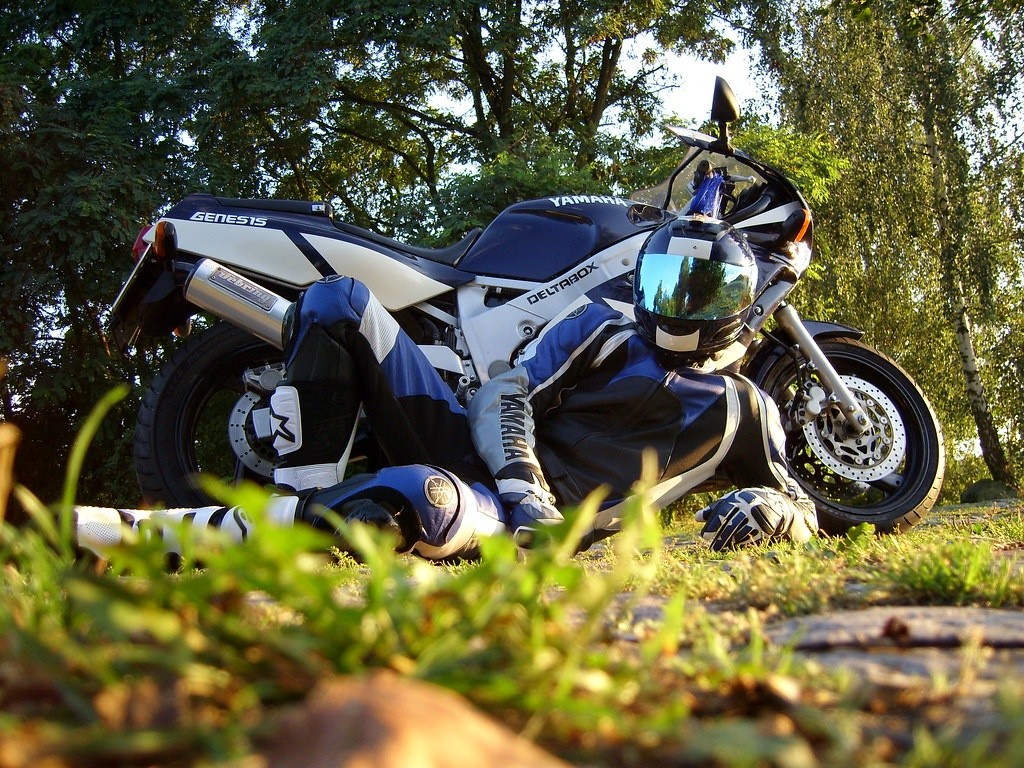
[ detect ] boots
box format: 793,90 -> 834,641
270,380 -> 363,493
71,488 -> 315,573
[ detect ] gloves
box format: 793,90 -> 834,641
695,487 -> 798,553
497,467 -> 565,541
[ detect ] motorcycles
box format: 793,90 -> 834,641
104,76 -> 947,536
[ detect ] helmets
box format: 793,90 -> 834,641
633,214 -> 758,365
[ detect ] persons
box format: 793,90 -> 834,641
48,216 -> 822,559
